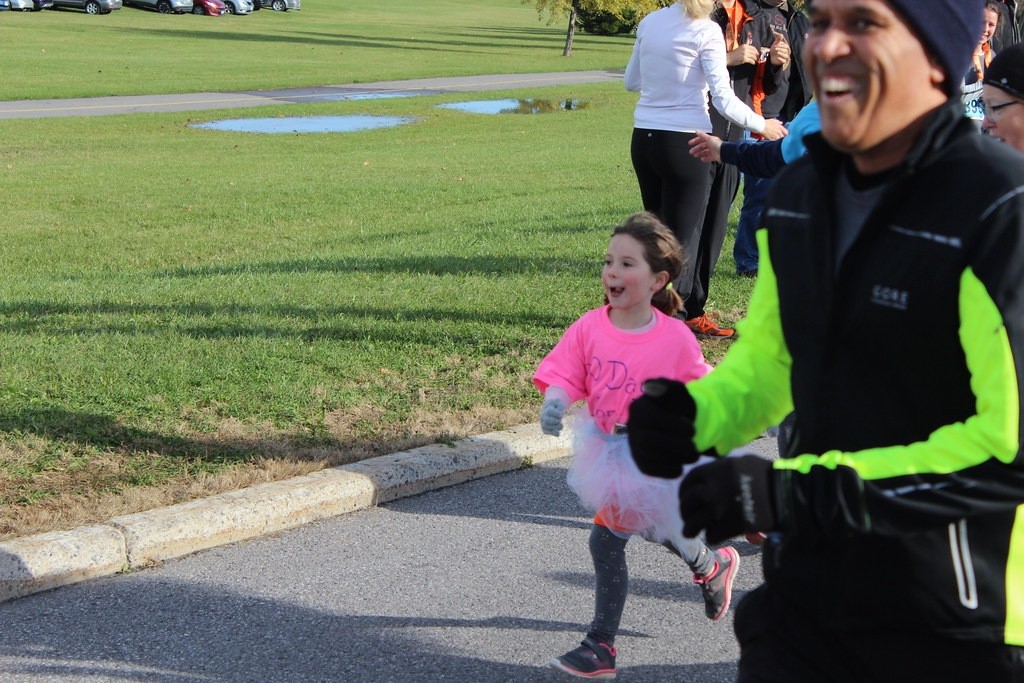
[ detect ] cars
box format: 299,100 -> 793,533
193,0 -> 227,16
0,0 -> 55,11
126,0 -> 194,13
259,0 -> 302,12
224,0 -> 254,15
56,0 -> 123,14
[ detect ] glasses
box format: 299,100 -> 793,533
975,98 -> 1017,118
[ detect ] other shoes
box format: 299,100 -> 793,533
736,268 -> 757,279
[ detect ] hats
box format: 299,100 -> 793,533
885,1 -> 983,98
983,44 -> 1024,100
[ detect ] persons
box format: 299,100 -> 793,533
533,213 -> 741,679
627,0 -> 1024,683
623,0 -> 1024,337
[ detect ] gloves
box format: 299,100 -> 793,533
542,395 -> 565,436
678,456 -> 780,548
626,384 -> 698,478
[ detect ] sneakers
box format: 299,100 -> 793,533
686,314 -> 735,339
547,637 -> 617,680
694,545 -> 740,620
